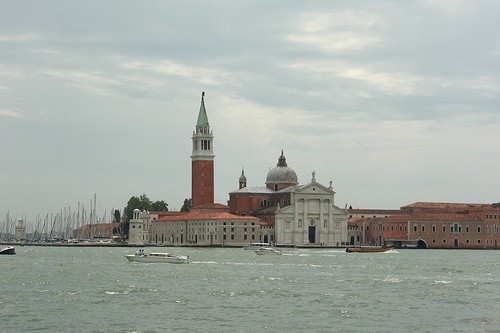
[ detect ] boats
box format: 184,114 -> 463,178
243,243 -> 273,250
345,246 -> 392,252
0,246 -> 16,255
255,248 -> 281,255
125,249 -> 190,264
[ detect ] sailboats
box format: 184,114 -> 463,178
1,193 -> 127,245
